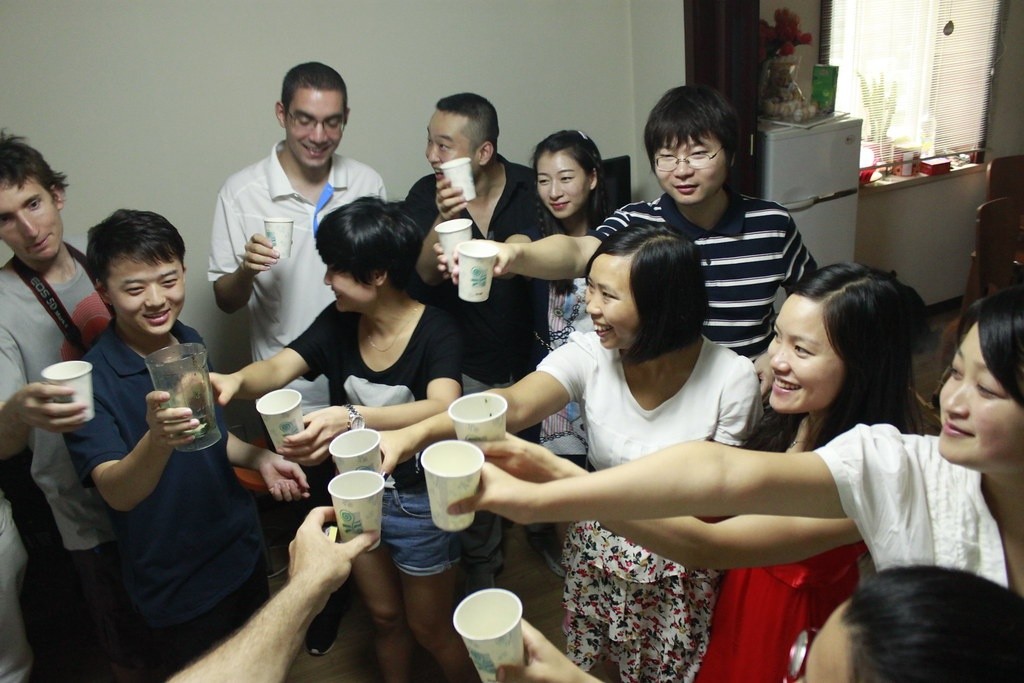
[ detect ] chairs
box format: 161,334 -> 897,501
965,157 -> 1024,311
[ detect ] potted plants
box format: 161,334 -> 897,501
850,71 -> 895,163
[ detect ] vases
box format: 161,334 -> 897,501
759,53 -> 801,110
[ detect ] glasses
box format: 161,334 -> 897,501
783,628 -> 816,683
653,145 -> 724,171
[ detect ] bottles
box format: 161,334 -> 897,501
918,109 -> 937,159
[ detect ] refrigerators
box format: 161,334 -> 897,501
757,116 -> 863,269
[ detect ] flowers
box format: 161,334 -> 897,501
759,7 -> 812,55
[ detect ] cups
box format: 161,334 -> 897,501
439,157 -> 476,203
264,218 -> 294,260
41,361 -> 95,421
143,343 -> 222,453
329,428 -> 381,475
453,589 -> 526,683
455,240 -> 499,302
255,389 -> 304,450
447,392 -> 507,446
329,470 -> 385,553
420,439 -> 485,532
434,219 -> 473,273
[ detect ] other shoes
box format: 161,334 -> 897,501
305,580 -> 350,655
528,527 -> 566,577
469,550 -> 503,593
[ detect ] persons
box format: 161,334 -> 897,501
472,261 -> 930,682
180,197 -> 481,683
0,135 -> 117,683
450,85 -> 816,433
448,286 -> 1024,594
495,566 -> 1024,683
64,208 -> 311,682
434,130 -> 612,472
162,506 -> 380,683
405,92 -> 567,577
208,62 -> 387,656
331,222 -> 763,683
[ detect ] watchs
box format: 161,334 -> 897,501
342,402 -> 365,431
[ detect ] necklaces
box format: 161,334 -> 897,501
789,417 -> 816,449
367,300 -> 419,353
